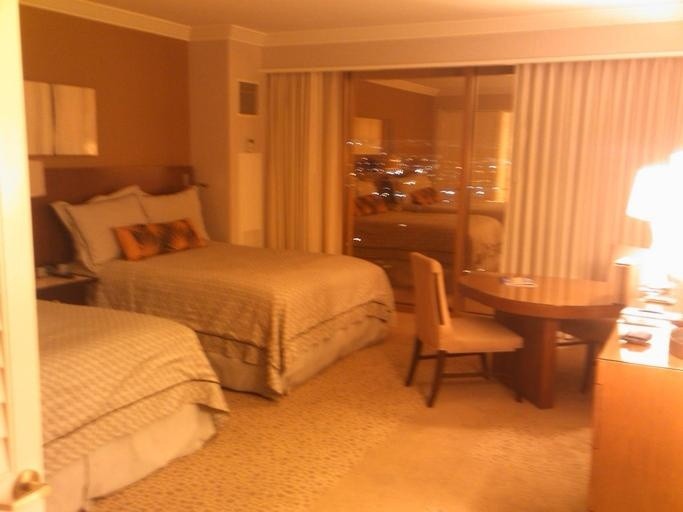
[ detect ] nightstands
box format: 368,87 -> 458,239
37,275 -> 98,305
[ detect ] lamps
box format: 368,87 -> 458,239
29,160 -> 46,198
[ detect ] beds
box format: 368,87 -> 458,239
38,299 -> 230,512
405,202 -> 503,225
355,211 -> 501,293
31,166 -> 394,402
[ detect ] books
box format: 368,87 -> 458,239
499,275 -> 538,288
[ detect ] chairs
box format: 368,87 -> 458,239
404,253 -> 526,408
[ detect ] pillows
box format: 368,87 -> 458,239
49,186 -> 210,273
355,174 -> 444,218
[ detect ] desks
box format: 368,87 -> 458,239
588,307 -> 683,512
